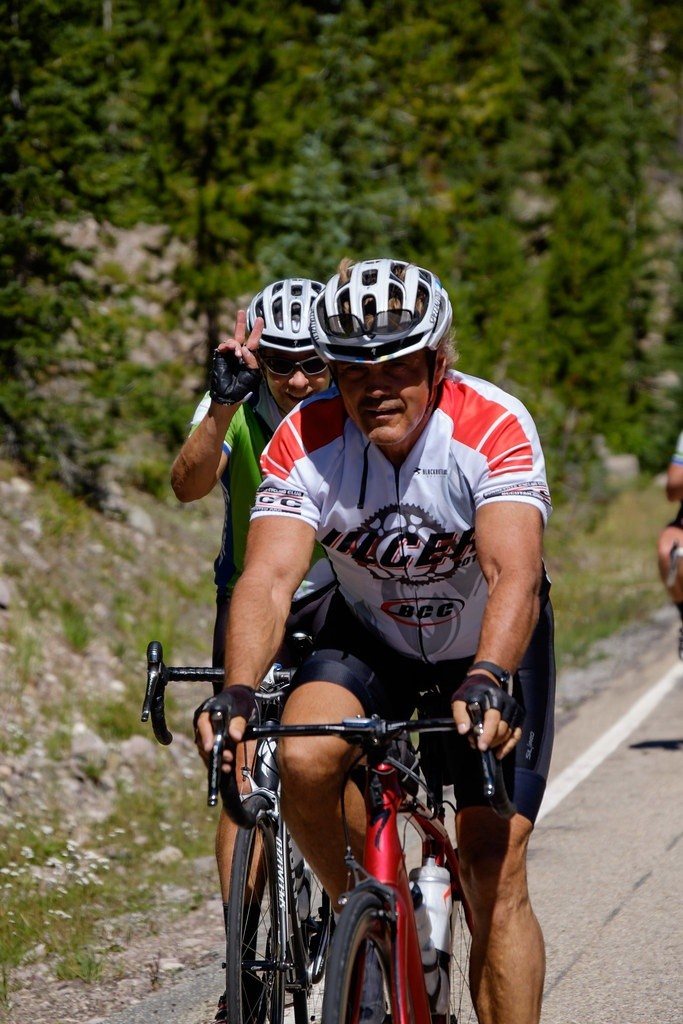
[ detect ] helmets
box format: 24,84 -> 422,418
245,277 -> 326,353
309,258 -> 453,373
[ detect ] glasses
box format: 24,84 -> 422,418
323,309 -> 417,336
260,355 -> 328,376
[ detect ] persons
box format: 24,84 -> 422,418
193,260 -> 558,1024
657,430 -> 683,659
170,279 -> 342,1024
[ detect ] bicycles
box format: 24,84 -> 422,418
138,640 -> 508,1024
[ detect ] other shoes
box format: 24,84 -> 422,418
214,975 -> 261,1024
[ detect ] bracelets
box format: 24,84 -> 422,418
469,662 -> 510,692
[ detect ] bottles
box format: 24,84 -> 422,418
406,879 -> 438,998
409,855 -> 453,1013
